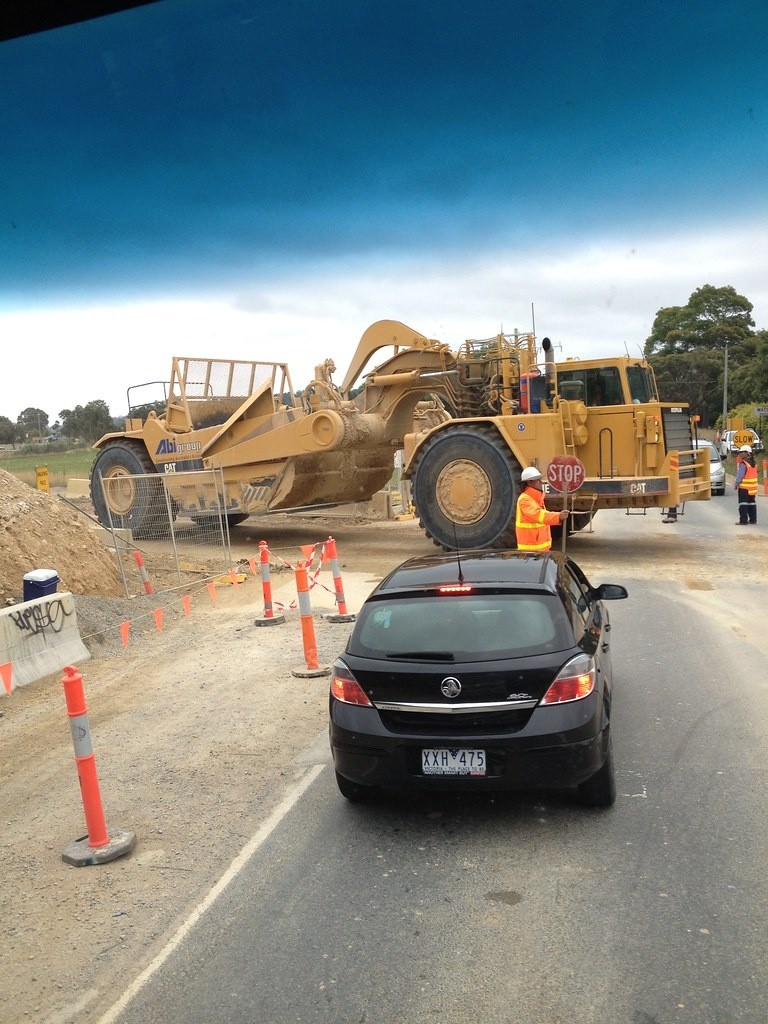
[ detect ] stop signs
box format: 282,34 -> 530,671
547,455 -> 585,494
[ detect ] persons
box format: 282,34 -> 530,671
733,445 -> 758,525
514,466 -> 570,552
662,507 -> 677,523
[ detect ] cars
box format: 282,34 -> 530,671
328,548 -> 629,811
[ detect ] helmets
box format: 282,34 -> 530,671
519,467 -> 544,484
737,445 -> 752,453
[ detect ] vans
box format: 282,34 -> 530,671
691,439 -> 728,496
721,428 -> 765,457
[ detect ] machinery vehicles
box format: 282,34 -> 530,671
88,302 -> 713,555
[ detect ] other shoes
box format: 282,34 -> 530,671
748,521 -> 757,524
735,522 -> 748,525
662,517 -> 678,523
